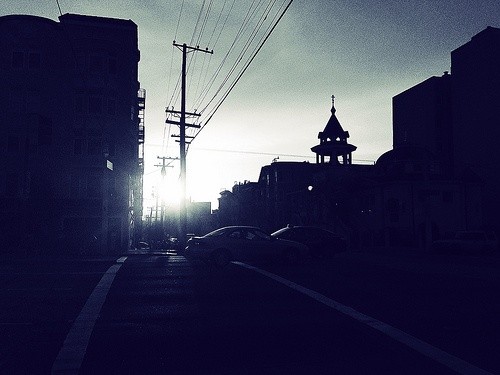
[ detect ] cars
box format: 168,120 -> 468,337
185,226 -> 308,269
134,232 -> 196,251
269,224 -> 347,257
